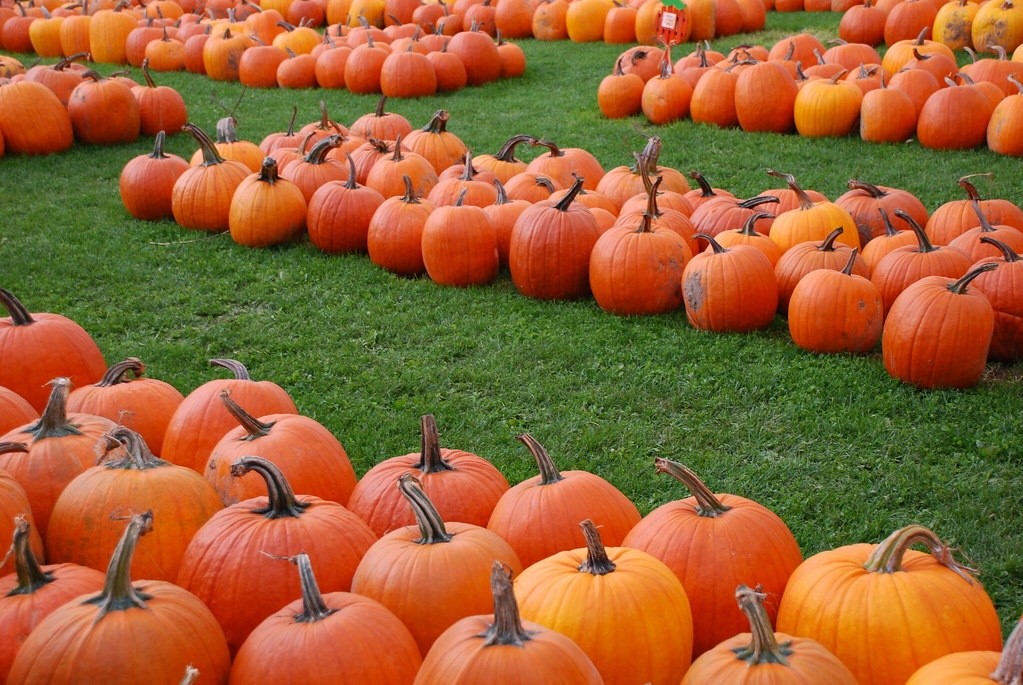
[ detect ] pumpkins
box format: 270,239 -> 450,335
0,0 -> 1023,389
0,287 -> 1023,685
